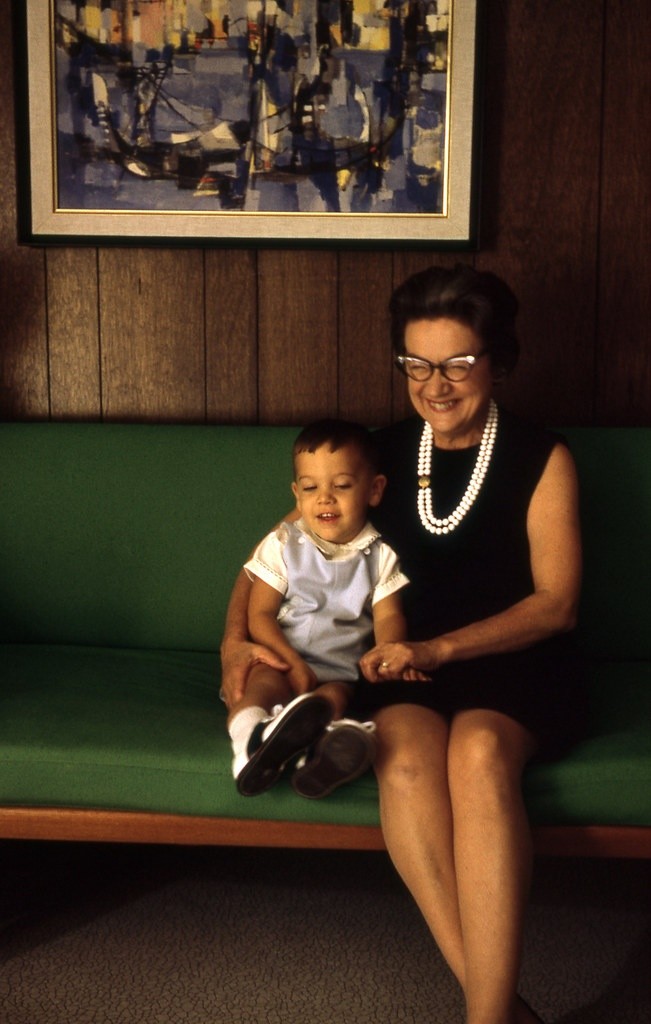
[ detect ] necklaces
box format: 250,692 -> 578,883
417,398 -> 498,535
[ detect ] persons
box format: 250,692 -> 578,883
222,267 -> 582,1024
226,415 -> 426,795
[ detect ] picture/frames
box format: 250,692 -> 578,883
9,0 -> 504,253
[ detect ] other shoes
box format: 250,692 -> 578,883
235,692 -> 334,799
290,718 -> 376,798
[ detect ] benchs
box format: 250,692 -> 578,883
0,421 -> 650,862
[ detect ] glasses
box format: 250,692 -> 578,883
394,345 -> 496,382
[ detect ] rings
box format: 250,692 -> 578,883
380,662 -> 387,666
